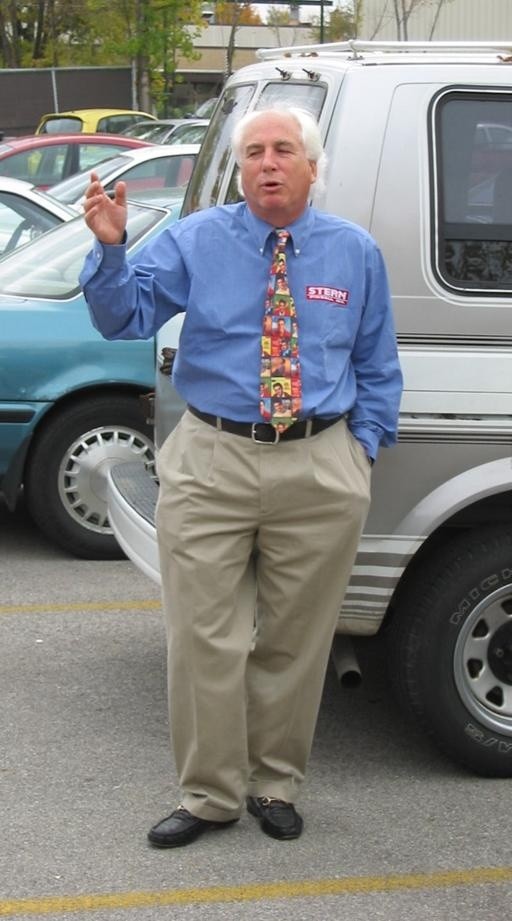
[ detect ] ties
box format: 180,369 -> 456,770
258,230 -> 301,434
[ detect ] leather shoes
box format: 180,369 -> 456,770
147,804 -> 240,848
246,796 -> 303,839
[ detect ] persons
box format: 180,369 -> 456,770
81,101 -> 404,850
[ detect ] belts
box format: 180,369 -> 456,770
187,405 -> 340,442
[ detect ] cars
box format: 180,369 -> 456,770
27,109 -> 160,179
1,174 -> 82,250
42,145 -> 205,212
183,96 -> 220,119
116,118 -> 210,148
3,184 -> 198,563
1,133 -> 198,193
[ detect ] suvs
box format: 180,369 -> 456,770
104,35 -> 511,780
